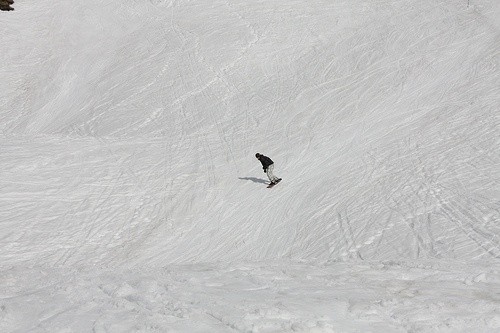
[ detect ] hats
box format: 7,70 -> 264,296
255,153 -> 260,157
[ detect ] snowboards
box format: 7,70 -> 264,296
267,178 -> 282,188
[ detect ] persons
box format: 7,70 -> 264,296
255,152 -> 281,185
